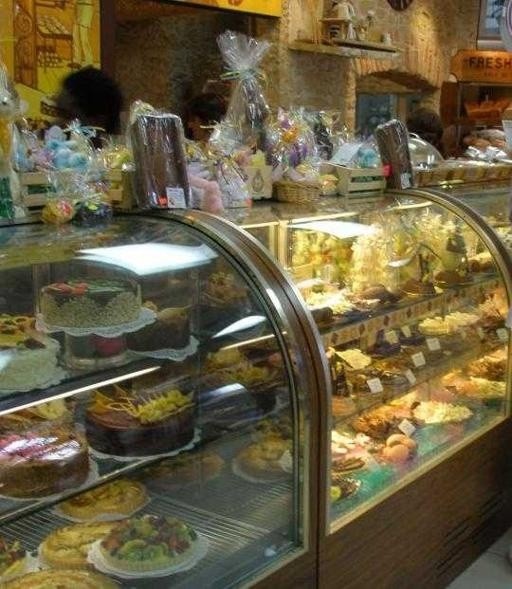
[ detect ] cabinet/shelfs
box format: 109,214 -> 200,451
1,202 -> 322,587
396,174 -> 511,416
127,186 -> 512,587
439,78 -> 512,157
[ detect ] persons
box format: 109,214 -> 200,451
403,106 -> 444,147
179,89 -> 229,141
36,64 -> 124,150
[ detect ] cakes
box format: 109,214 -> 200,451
1,538 -> 32,583
324,290 -> 508,418
329,346 -> 506,505
194,348 -> 280,431
84,382 -> 198,458
2,569 -> 122,589
86,514 -> 209,580
34,521 -> 130,570
230,433 -> 296,484
193,269 -> 259,332
141,452 -> 227,489
1,398 -> 102,498
286,231 -> 499,326
0,275 -> 199,398
52,477 -> 152,523
